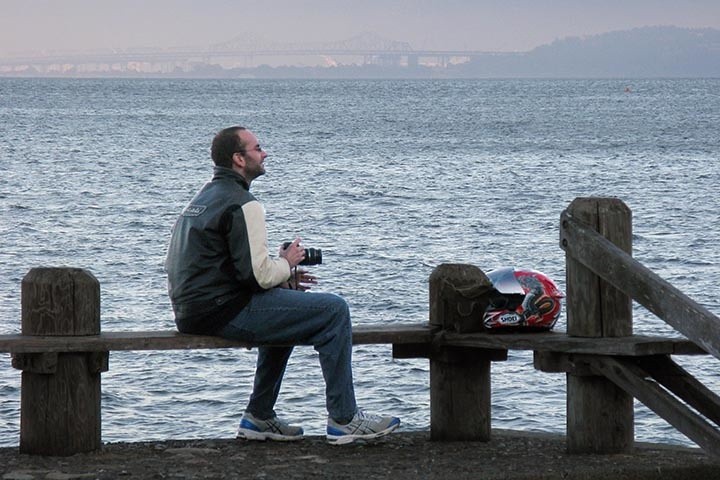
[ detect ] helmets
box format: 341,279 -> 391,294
481,266 -> 567,332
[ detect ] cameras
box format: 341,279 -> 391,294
283,242 -> 322,265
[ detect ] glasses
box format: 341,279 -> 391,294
233,145 -> 262,153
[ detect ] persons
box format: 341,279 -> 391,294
165,126 -> 400,445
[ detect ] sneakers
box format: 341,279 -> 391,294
236,413 -> 304,442
326,408 -> 400,445
